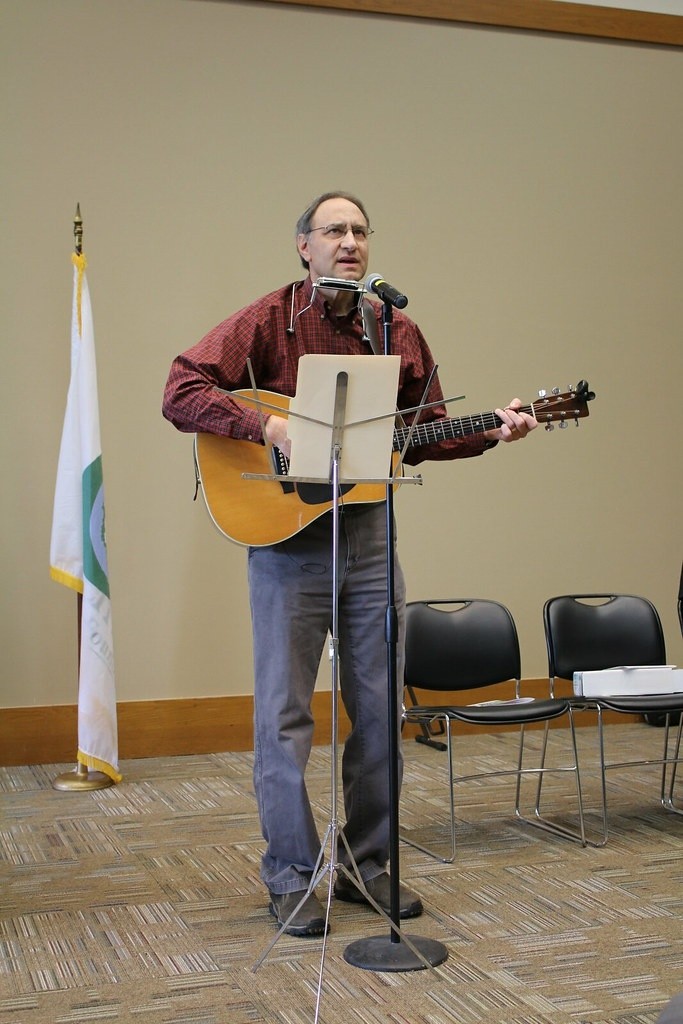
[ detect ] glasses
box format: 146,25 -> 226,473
305,223 -> 374,238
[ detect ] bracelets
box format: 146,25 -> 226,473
486,441 -> 494,447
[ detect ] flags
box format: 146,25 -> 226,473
50,252 -> 118,781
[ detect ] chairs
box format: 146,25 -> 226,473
533,592 -> 682,848
398,597 -> 588,862
666,600 -> 683,809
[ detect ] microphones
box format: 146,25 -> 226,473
364,273 -> 409,309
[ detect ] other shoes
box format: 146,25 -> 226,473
334,872 -> 423,920
268,888 -> 331,937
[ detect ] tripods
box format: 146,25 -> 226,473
238,354 -> 445,1024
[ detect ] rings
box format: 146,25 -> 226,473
512,428 -> 516,432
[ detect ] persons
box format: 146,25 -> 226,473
162,191 -> 537,936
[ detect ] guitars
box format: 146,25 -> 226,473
193,379 -> 596,549
